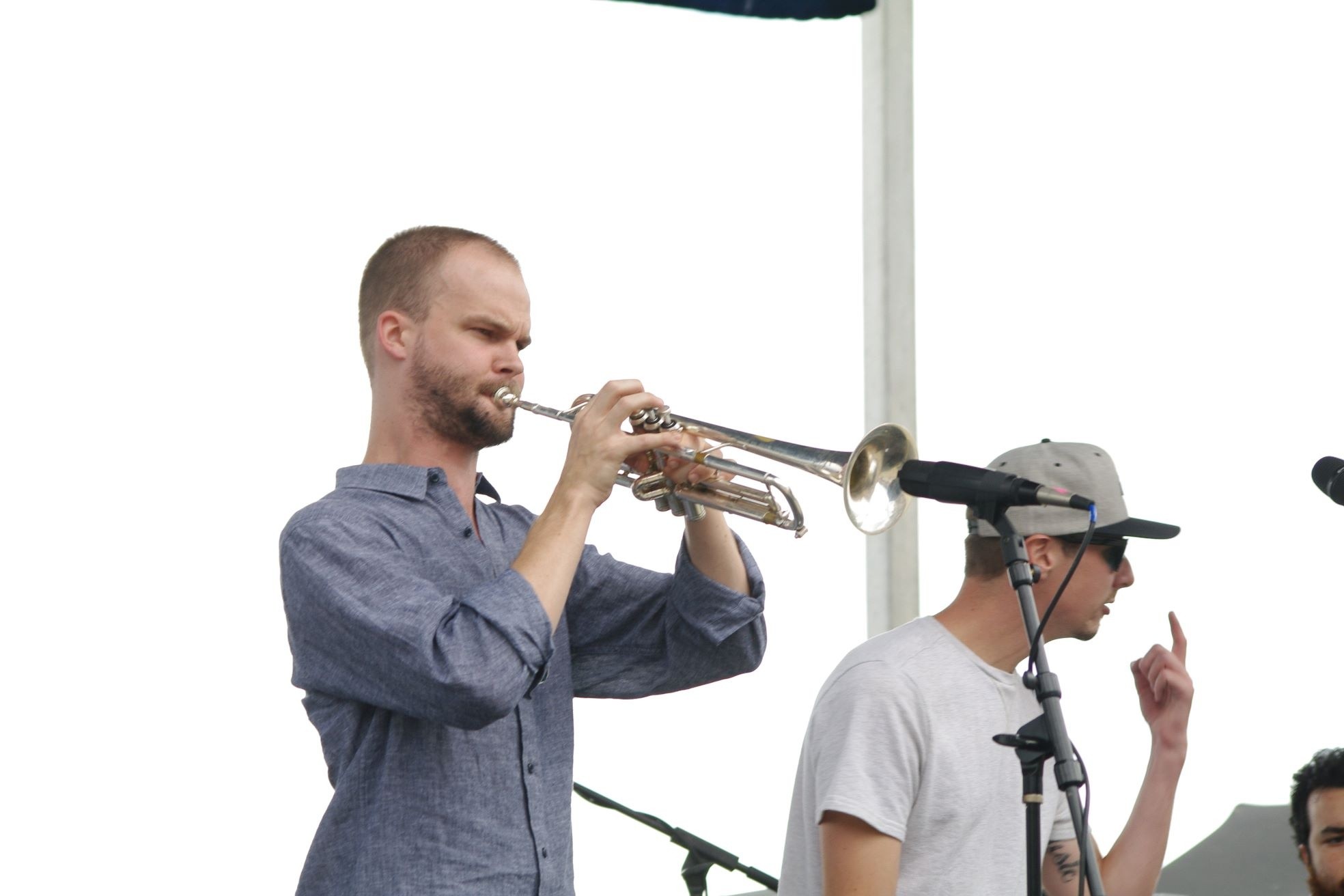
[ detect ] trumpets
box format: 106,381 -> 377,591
495,383 -> 916,538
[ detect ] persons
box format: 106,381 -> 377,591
1289,747 -> 1344,896
277,224 -> 765,896
775,437 -> 1194,896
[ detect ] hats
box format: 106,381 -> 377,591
964,439 -> 1181,540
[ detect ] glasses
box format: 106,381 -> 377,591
1061,537 -> 1129,570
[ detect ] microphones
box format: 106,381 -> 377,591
1311,456 -> 1344,506
898,459 -> 1095,511
